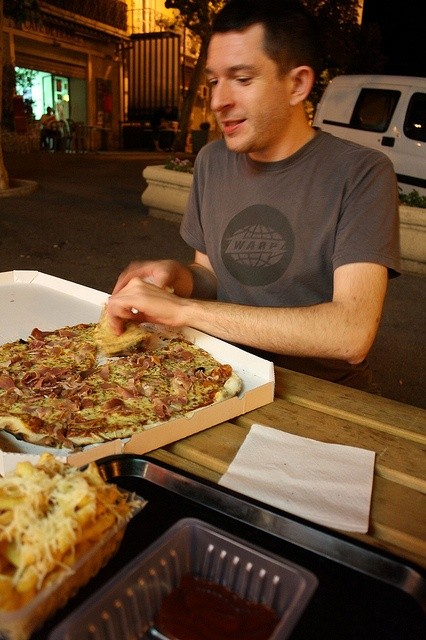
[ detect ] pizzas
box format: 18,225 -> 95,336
0,319 -> 240,447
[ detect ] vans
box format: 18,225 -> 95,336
310,73 -> 426,201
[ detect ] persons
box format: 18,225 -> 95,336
106,0 -> 403,395
40,106 -> 57,151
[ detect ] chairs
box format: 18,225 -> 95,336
47,118 -> 110,153
